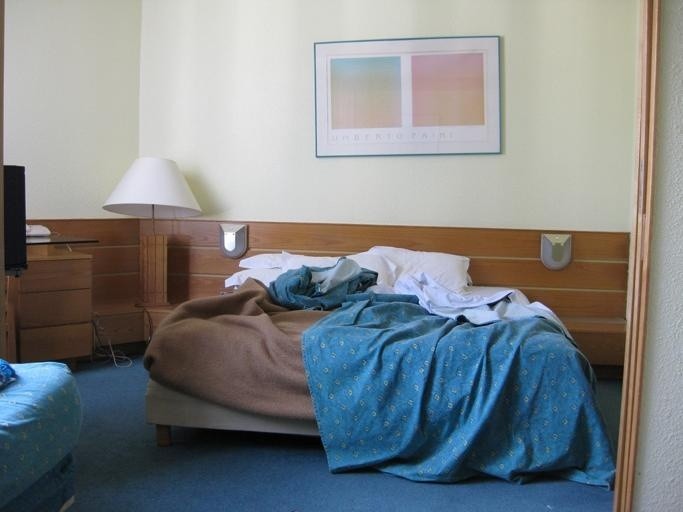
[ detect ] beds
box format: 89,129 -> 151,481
143,244 -> 622,492
0,357 -> 84,512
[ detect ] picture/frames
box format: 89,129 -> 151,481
311,34 -> 506,158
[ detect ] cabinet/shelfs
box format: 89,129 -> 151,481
13,252 -> 95,370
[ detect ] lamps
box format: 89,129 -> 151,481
101,156 -> 203,305
